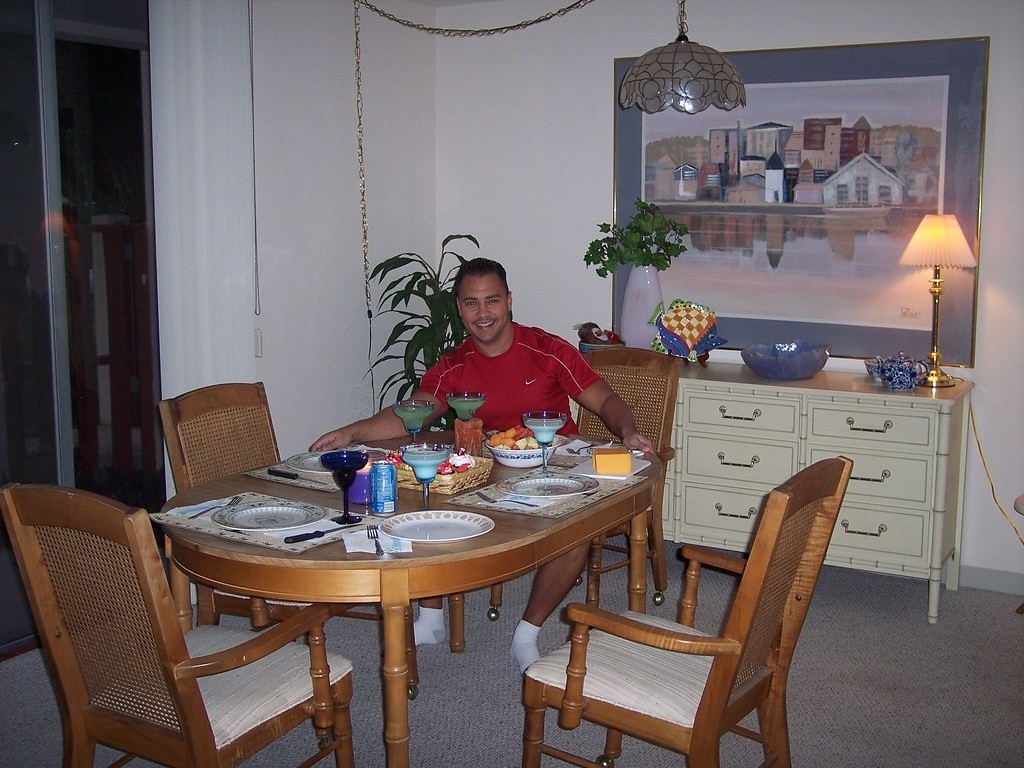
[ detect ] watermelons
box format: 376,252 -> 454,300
511,425 -> 541,450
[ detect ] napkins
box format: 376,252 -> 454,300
342,529 -> 415,555
554,439 -> 613,456
165,500 -> 227,521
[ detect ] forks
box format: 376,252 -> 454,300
566,444 -> 596,454
366,525 -> 384,556
188,496 -> 243,520
476,491 -> 540,508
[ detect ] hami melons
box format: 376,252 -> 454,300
489,427 -> 515,448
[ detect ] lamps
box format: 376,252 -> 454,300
618,2 -> 748,116
895,214 -> 978,389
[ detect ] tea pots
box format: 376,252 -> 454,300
875,351 -> 929,392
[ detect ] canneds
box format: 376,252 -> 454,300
368,460 -> 397,517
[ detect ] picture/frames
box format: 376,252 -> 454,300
611,37 -> 990,368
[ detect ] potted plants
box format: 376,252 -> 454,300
585,198 -> 689,351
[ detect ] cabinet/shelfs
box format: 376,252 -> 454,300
659,381 -> 965,581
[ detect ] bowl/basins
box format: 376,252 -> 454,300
485,435 -> 566,467
742,341 -> 832,379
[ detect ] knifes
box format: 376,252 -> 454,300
284,524 -> 365,543
268,468 -> 328,487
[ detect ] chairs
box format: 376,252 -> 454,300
0,479 -> 360,767
523,454 -> 854,768
159,381 -> 304,632
488,348 -> 683,621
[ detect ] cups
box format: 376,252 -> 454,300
445,391 -> 486,421
864,359 -> 878,379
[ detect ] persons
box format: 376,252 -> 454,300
308,258 -> 657,678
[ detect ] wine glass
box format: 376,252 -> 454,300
399,443 -> 451,511
522,410 -> 567,474
320,451 -> 368,524
391,400 -> 433,444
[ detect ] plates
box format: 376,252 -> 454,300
494,472 -> 599,497
212,500 -> 327,532
286,448 -> 385,474
580,341 -> 626,348
566,456 -> 653,480
378,510 -> 495,543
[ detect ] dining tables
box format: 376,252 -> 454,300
159,427 -> 661,767
